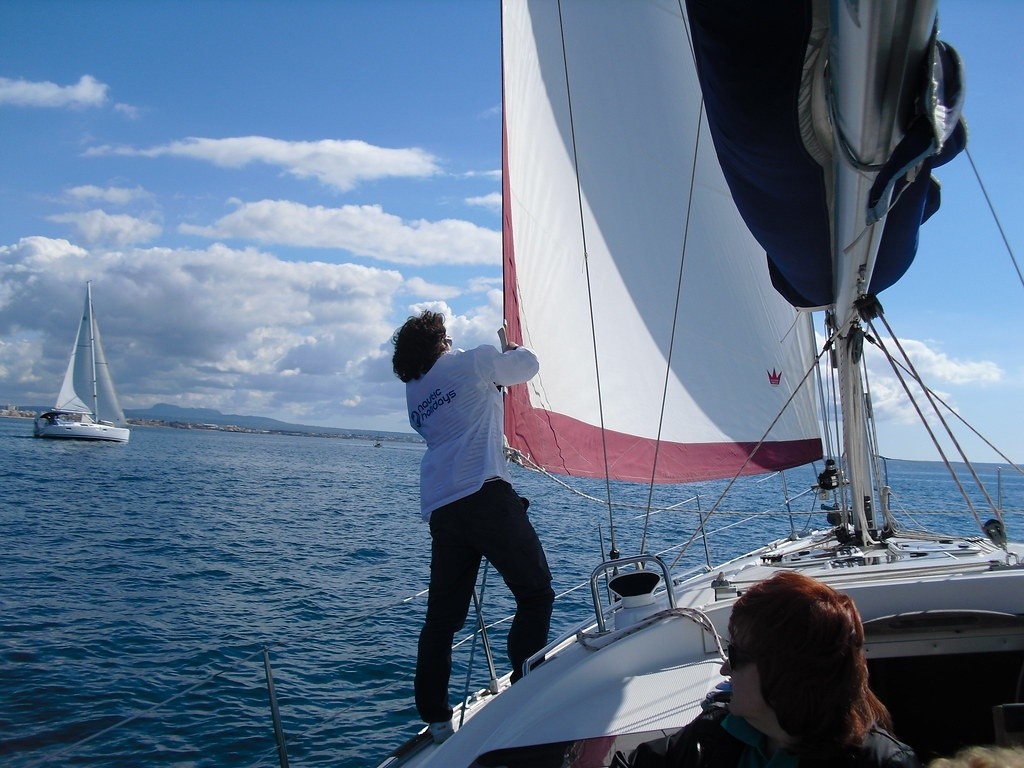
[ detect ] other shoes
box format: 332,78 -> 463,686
428,721 -> 455,744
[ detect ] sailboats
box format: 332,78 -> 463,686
33,280 -> 131,443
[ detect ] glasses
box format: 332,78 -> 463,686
441,335 -> 453,348
725,645 -> 776,673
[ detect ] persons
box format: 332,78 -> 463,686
613,570 -> 923,768
394,311 -> 558,745
